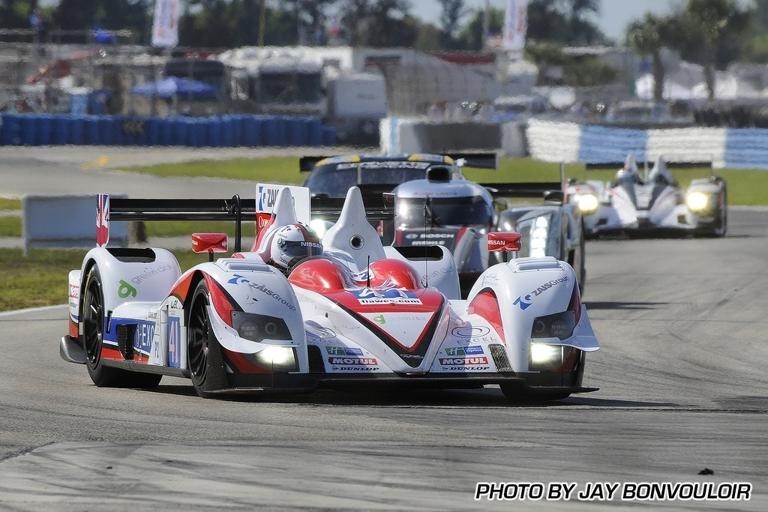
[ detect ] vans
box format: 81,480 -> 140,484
84,46 -> 389,144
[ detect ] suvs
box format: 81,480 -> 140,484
486,92 -> 550,115
600,94 -> 670,124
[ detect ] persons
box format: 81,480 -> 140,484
614,167 -> 638,184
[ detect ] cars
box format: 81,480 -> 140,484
556,156 -> 729,242
